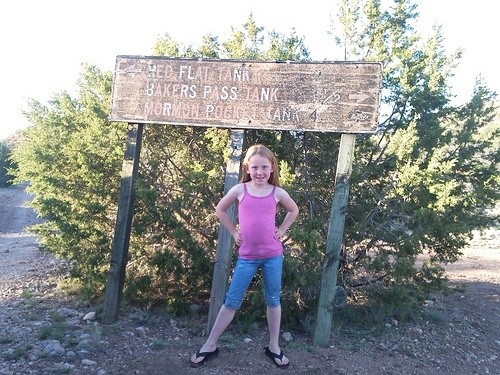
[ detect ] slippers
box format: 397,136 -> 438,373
190,348 -> 218,368
266,347 -> 290,368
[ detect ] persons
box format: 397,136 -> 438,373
190,144 -> 300,366
